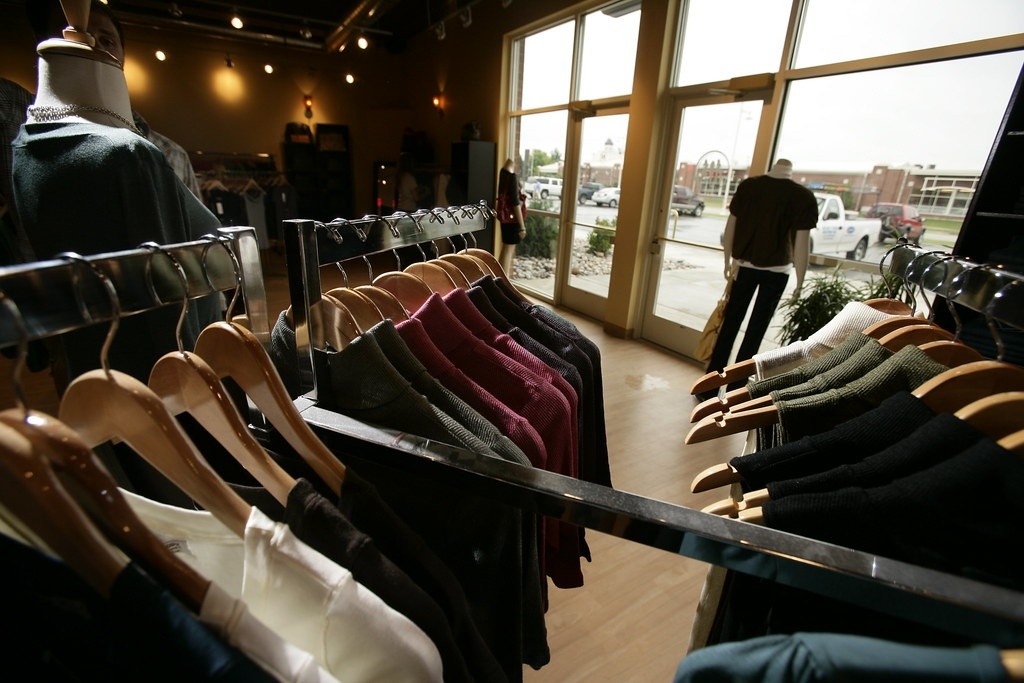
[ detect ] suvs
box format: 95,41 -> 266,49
868,202 -> 928,248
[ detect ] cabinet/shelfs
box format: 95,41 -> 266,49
450,139 -> 497,259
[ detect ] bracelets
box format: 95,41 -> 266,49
520,228 -> 527,231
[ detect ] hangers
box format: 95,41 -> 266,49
0,166 -> 601,683
676,242 -> 1024,683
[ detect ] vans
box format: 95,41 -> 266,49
524,175 -> 563,201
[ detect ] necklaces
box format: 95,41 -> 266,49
27,105 -> 149,138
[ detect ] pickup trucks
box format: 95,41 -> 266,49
805,191 -> 883,263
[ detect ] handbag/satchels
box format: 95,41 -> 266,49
692,271 -> 734,361
497,173 -> 528,225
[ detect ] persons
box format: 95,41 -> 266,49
497,158 -> 529,274
12,39 -> 222,401
85,0 -> 203,204
695,158 -> 818,403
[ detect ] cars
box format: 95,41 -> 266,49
592,187 -> 621,209
577,181 -> 605,205
670,184 -> 705,218
